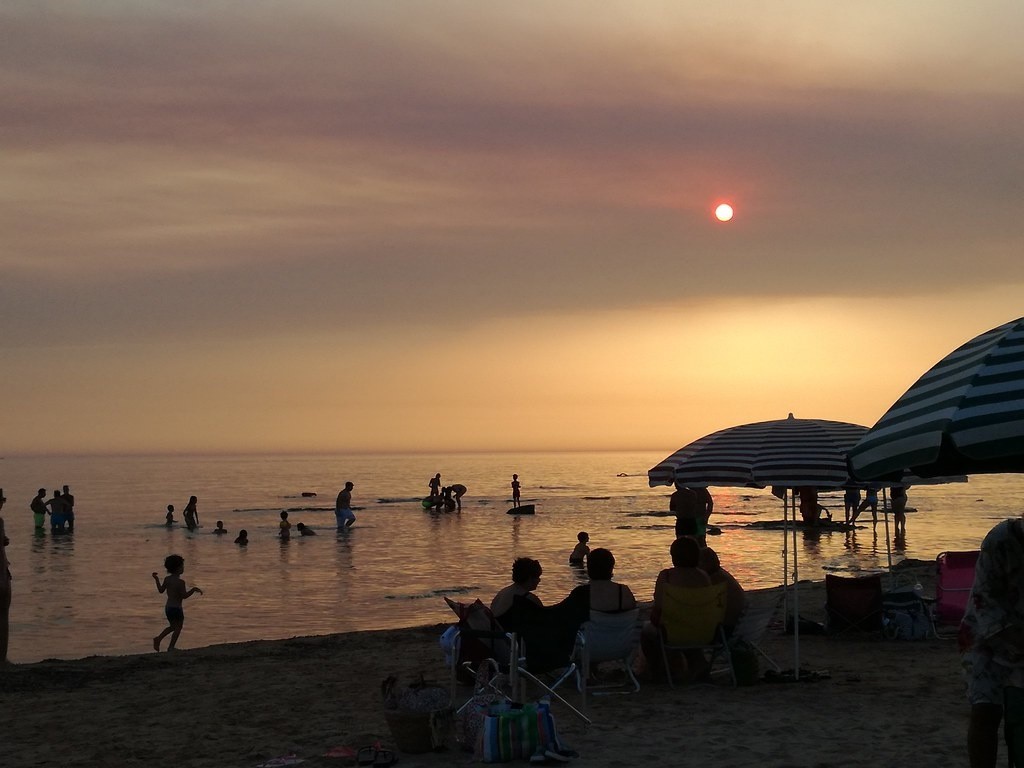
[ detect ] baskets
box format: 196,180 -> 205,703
383,707 -> 457,754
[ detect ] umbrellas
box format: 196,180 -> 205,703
648,413 -> 968,681
846,317 -> 1024,480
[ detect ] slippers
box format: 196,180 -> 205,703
321,745 -> 354,758
357,744 -> 377,765
256,753 -> 306,768
529,754 -> 548,762
373,748 -> 400,768
545,747 -> 581,761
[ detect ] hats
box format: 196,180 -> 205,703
439,625 -> 461,665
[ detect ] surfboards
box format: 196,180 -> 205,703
507,505 -> 535,514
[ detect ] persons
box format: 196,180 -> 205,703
422,472 -> 747,691
30,485 -> 316,541
152,554 -> 203,653
335,482 -> 355,530
0,488 -> 16,669
957,518 -> 1024,768
794,485 -> 910,534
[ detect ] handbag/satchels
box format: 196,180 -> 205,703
464,658 -> 512,750
478,695 -> 560,764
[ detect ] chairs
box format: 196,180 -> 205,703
825,549 -> 981,638
439,582 -> 794,725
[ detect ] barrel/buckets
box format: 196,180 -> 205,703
386,709 -> 434,752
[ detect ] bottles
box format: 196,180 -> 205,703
914,581 -> 923,594
